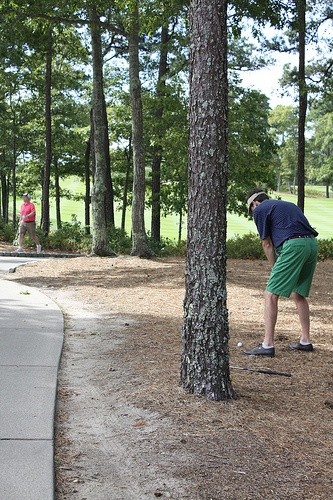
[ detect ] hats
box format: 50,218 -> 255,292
21,193 -> 30,198
247,191 -> 267,215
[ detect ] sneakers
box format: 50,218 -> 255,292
37,244 -> 41,253
291,340 -> 313,351
15,249 -> 23,252
243,342 -> 276,356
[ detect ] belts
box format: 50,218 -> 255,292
289,235 -> 314,239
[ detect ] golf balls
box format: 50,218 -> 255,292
237,342 -> 243,347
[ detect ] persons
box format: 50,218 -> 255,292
14,192 -> 42,255
242,190 -> 320,356
